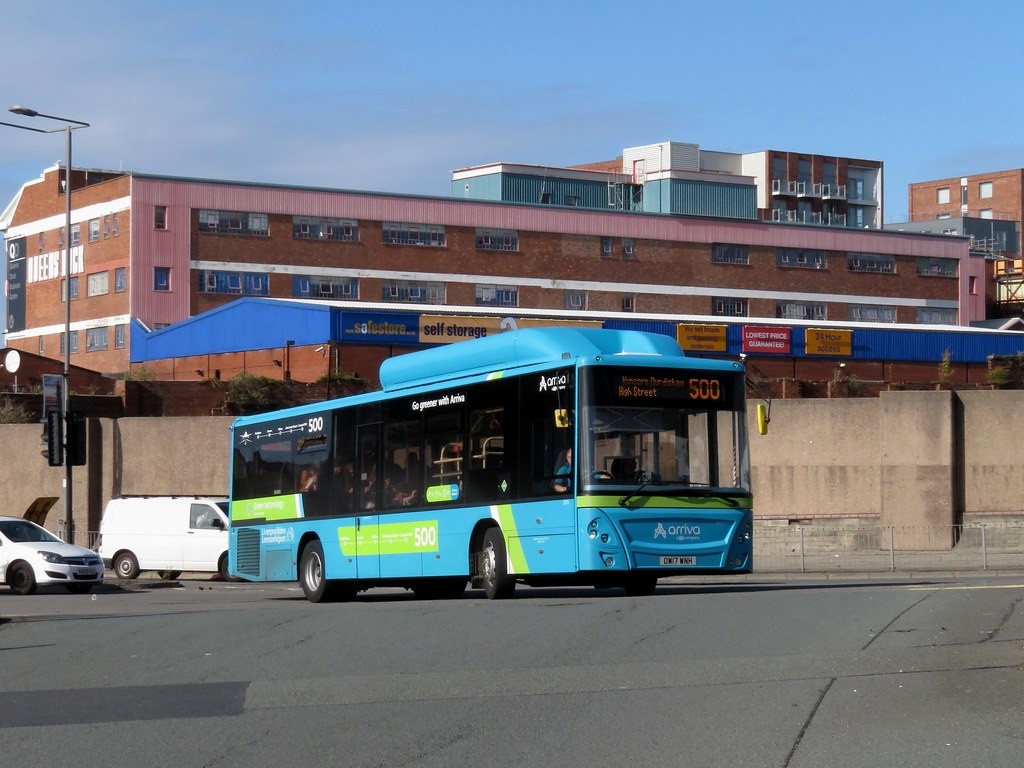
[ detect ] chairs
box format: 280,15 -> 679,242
609,455 -> 640,482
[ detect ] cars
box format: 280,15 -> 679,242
0,514 -> 104,596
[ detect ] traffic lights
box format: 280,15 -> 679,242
39,408 -> 62,468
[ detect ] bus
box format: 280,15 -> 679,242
226,327 -> 771,604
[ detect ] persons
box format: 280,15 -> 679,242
300,445 -> 420,511
553,448 -> 610,493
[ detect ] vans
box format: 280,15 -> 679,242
99,493 -> 245,584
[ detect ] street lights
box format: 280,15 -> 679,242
1,103 -> 91,548
285,340 -> 295,380
